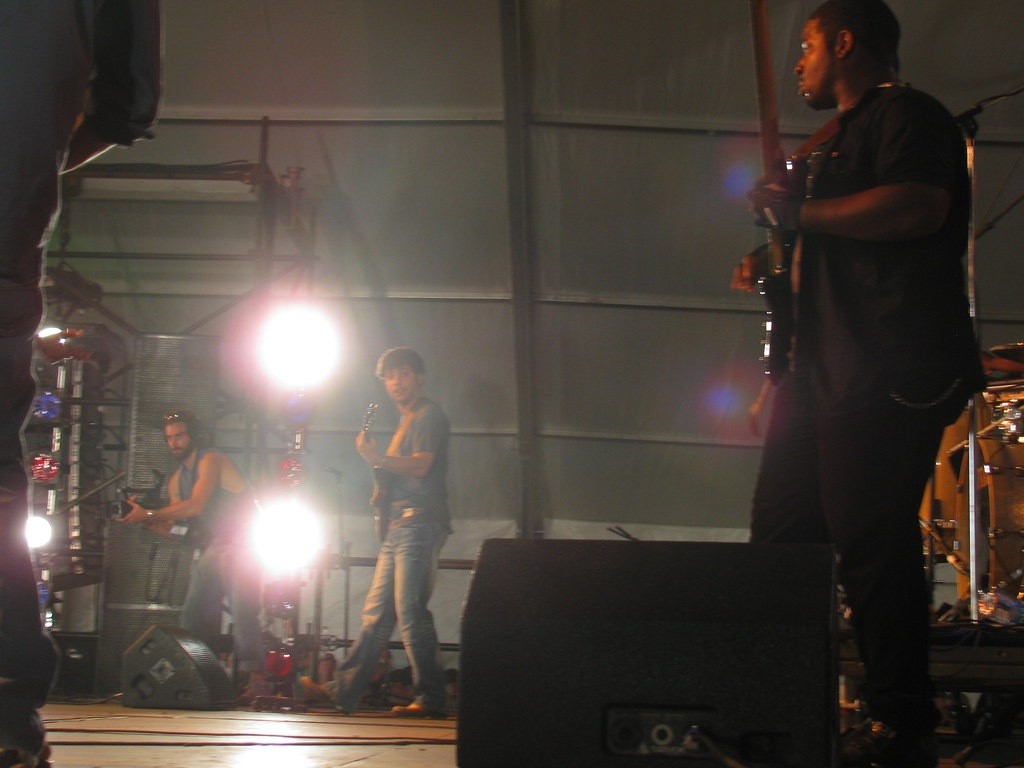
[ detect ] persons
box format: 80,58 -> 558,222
0,0 -> 165,768
301,346 -> 451,720
115,410 -> 277,705
729,0 -> 989,768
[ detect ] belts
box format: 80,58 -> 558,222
401,506 -> 423,517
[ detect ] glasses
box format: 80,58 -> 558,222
161,413 -> 184,423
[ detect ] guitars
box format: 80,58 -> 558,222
116,499 -> 191,548
747,0 -> 801,403
358,401 -> 392,545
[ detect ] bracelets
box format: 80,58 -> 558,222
147,510 -> 158,524
784,197 -> 805,232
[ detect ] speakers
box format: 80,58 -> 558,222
457,537 -> 840,768
96,335 -> 243,711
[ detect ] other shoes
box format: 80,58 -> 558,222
392,703 -> 447,720
837,721 -> 940,768
301,676 -> 348,714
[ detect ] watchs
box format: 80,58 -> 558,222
372,457 -> 387,475
